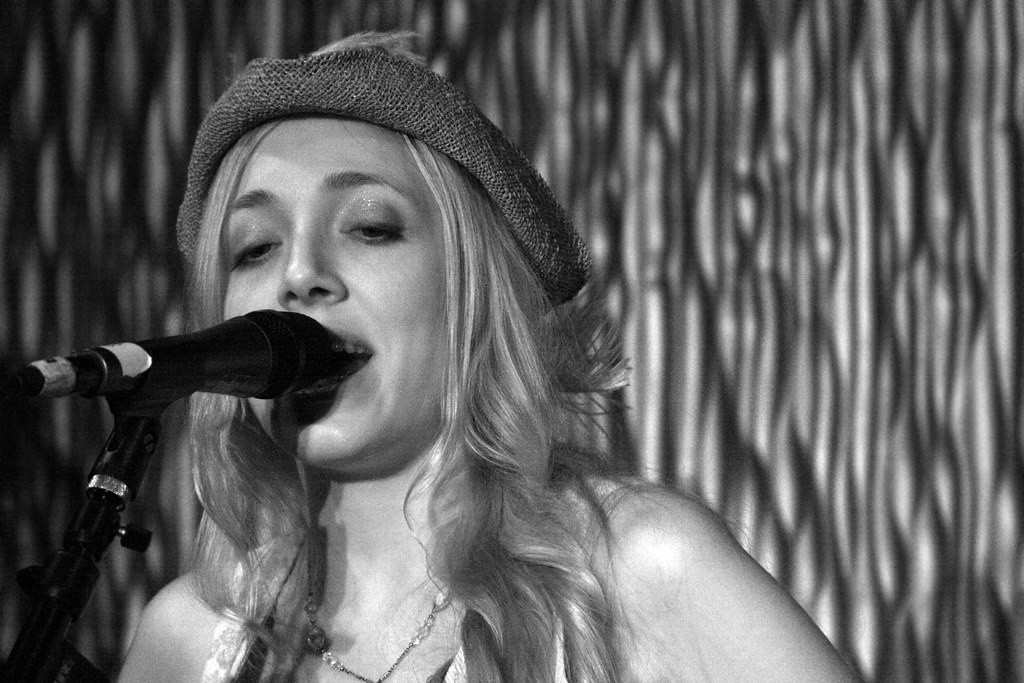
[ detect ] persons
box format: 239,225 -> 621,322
108,33 -> 861,683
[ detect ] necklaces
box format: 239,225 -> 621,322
300,481 -> 452,683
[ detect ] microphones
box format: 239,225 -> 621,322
17,309 -> 330,403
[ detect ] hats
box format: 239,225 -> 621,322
177,45 -> 592,307
